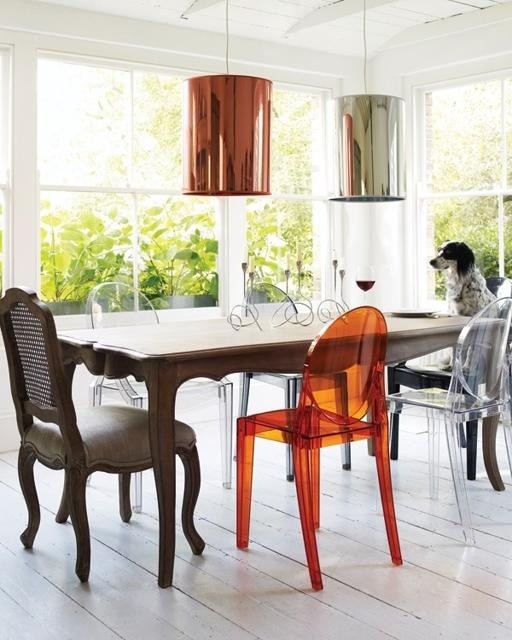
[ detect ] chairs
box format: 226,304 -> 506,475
239,284 -> 351,484
385,296 -> 511,545
235,305 -> 405,590
386,278 -> 511,482
0,277 -> 206,591
88,282 -> 234,514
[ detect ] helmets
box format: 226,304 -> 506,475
383,310 -> 437,317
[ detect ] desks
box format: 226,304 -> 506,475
58,311 -> 503,588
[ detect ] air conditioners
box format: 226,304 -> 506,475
428,238 -> 498,374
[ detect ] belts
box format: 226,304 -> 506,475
356,268 -> 376,306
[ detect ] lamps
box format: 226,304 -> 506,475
182,0 -> 272,195
328,0 -> 406,201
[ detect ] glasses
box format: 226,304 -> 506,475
328,0 -> 406,201
182,0 -> 272,195
